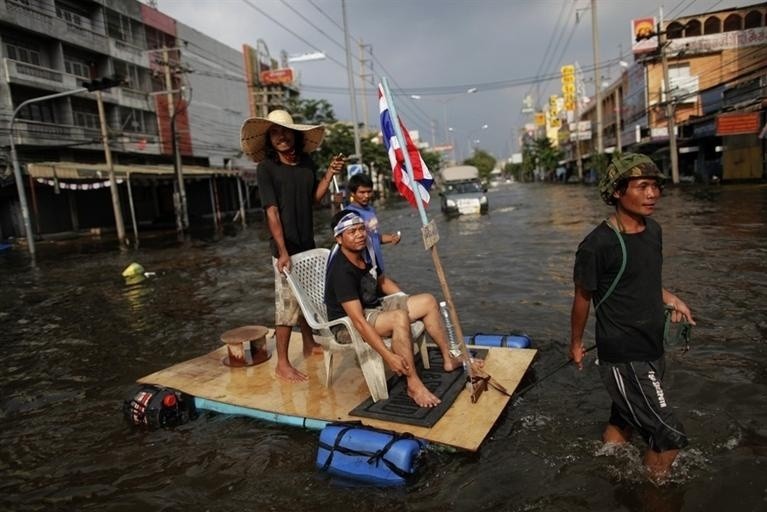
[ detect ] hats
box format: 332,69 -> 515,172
239,109 -> 326,164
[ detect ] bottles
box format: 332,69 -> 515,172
438,301 -> 458,350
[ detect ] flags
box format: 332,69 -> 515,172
375,79 -> 436,211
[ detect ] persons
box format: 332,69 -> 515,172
332,173 -> 403,280
566,147 -> 698,474
239,108 -> 350,385
319,206 -> 484,409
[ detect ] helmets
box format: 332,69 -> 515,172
599,151 -> 663,206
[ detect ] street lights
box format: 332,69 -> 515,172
409,87 -> 489,164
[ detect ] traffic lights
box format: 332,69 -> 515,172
81,73 -> 123,92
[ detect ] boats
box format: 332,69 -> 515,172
124,324 -> 538,485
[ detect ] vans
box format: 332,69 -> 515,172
439,166 -> 487,218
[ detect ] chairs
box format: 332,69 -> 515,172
279,246 -> 429,404
